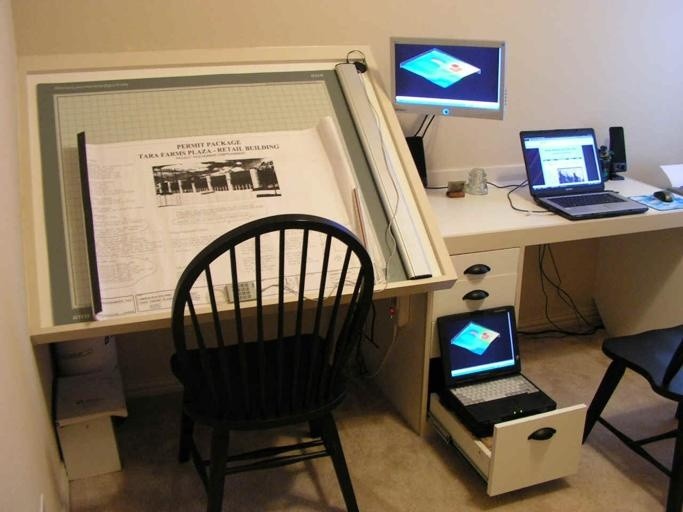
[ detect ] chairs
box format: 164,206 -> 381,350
582,324 -> 682,512
171,214 -> 374,510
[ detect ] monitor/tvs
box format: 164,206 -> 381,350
389,36 -> 506,121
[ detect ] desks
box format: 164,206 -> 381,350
357,178 -> 683,496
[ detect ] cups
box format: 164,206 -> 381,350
466,168 -> 488,195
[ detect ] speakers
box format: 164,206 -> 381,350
405,136 -> 428,188
608,127 -> 627,180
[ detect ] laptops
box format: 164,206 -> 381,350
437,304 -> 557,438
519,127 -> 649,222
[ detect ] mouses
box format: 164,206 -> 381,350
654,191 -> 673,203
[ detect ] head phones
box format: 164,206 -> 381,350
346,49 -> 369,72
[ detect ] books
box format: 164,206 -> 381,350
55,371 -> 129,429
